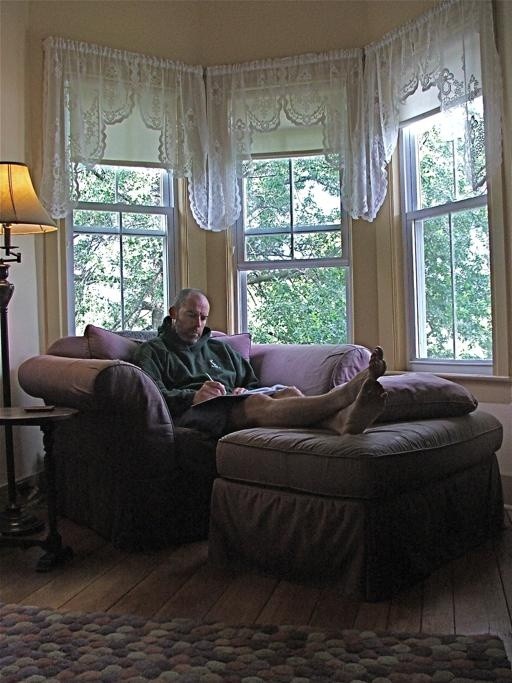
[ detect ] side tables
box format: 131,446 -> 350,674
0,407 -> 81,572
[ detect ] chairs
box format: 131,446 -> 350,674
17,325 -> 372,555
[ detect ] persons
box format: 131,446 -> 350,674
131,288 -> 391,435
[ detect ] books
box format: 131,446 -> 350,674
191,383 -> 288,409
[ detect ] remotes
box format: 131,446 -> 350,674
24,405 -> 54,412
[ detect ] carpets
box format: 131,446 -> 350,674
0,599 -> 512,682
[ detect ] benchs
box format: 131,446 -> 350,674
207,413 -> 505,601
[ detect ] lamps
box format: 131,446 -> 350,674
0,162 -> 58,539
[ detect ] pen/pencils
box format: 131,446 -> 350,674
205,372 -> 227,395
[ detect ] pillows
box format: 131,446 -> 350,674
376,371 -> 479,423
